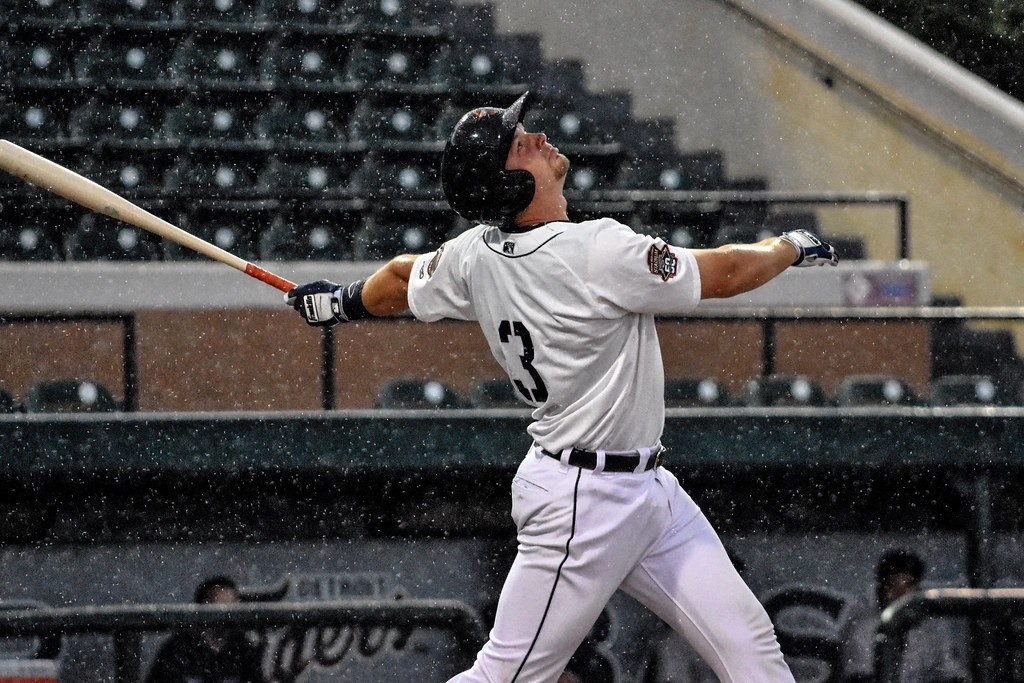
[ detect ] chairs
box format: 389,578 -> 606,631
470,379 -> 537,409
373,381 -> 468,409
0,390 -> 26,414
666,379 -> 741,408
26,379 -> 118,413
928,298 -> 1024,406
744,377 -> 835,408
0,0 -> 865,264
840,374 -> 926,407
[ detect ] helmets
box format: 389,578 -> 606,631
441,90 -> 535,230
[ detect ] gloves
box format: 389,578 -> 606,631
284,279 -> 380,327
780,228 -> 839,268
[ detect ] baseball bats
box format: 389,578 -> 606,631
0,140 -> 299,292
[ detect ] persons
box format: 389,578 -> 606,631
840,550 -> 971,683
284,89 -> 839,683
144,574 -> 270,683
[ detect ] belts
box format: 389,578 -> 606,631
541,446 -> 667,473
